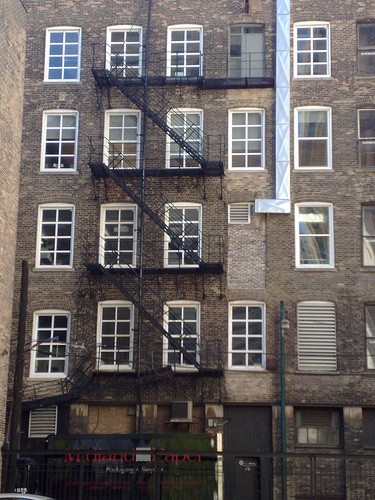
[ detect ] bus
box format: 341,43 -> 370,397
30,433 -> 217,500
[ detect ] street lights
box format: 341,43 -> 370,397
280,300 -> 288,500
4,337 -> 58,442
